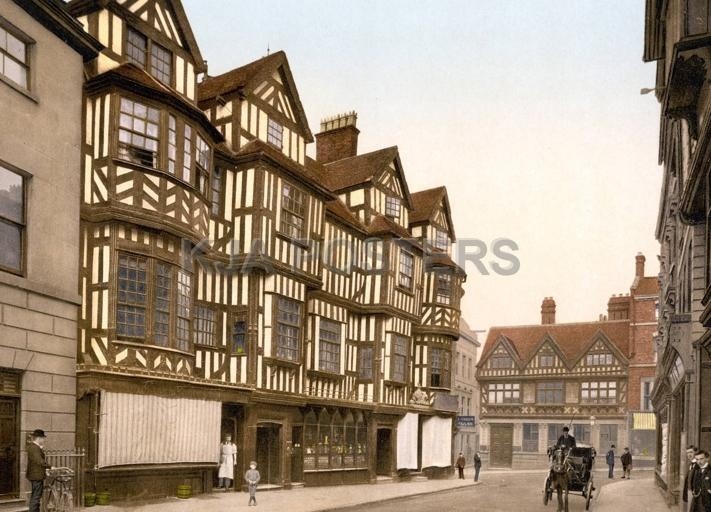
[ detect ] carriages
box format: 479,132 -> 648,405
543,441 -> 596,512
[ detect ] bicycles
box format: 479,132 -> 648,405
39,467 -> 75,512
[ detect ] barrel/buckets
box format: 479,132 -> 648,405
96,492 -> 111,505
177,484 -> 192,499
84,492 -> 97,506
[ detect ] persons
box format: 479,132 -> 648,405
690,450 -> 711,512
621,447 -> 632,479
27,430 -> 51,511
218,433 -> 237,492
456,452 -> 465,480
556,427 -> 576,456
474,451 -> 481,481
606,445 -> 616,478
684,446 -> 696,511
245,461 -> 261,506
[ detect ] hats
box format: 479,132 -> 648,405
561,426 -> 570,432
29,427 -> 47,439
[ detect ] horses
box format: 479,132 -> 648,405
551,444 -> 576,512
